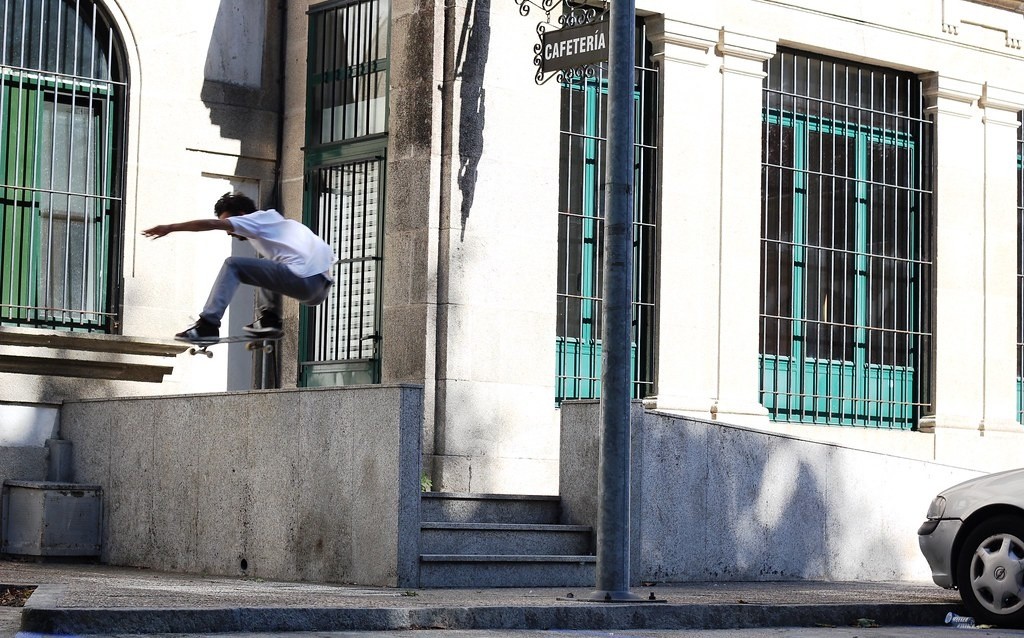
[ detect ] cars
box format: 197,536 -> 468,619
920,467 -> 1024,629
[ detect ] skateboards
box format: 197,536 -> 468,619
184,332 -> 286,358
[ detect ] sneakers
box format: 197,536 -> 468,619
242,317 -> 284,339
173,317 -> 221,342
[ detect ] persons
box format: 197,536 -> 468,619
141,193 -> 338,342
